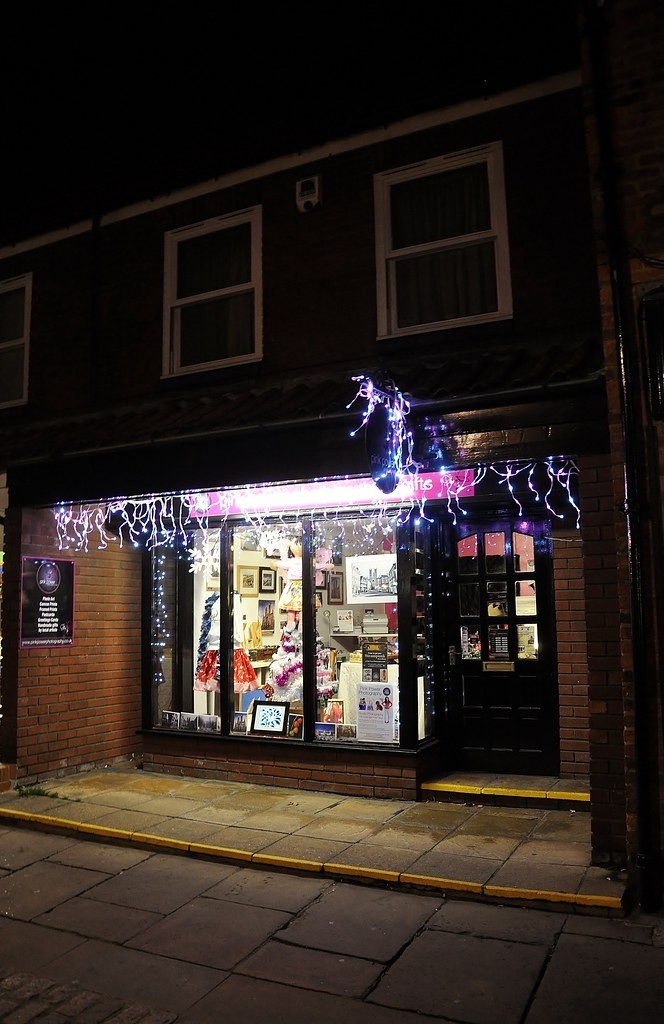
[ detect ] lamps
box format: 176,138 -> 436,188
192,493 -> 210,514
371,473 -> 400,493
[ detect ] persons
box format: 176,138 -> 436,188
271,537 -> 334,633
194,590 -> 260,693
359,698 -> 366,710
367,700 -> 373,710
382,697 -> 392,723
376,701 -> 383,711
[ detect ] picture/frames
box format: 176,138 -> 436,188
316,571 -> 326,590
263,548 -> 281,559
316,592 -> 323,608
259,566 -> 276,594
240,537 -> 262,552
326,571 -> 344,605
205,549 -> 220,591
237,565 -> 259,597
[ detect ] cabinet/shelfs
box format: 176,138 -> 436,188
329,633 -> 398,681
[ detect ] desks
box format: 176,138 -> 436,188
338,661 -> 425,740
205,659 -> 272,716
